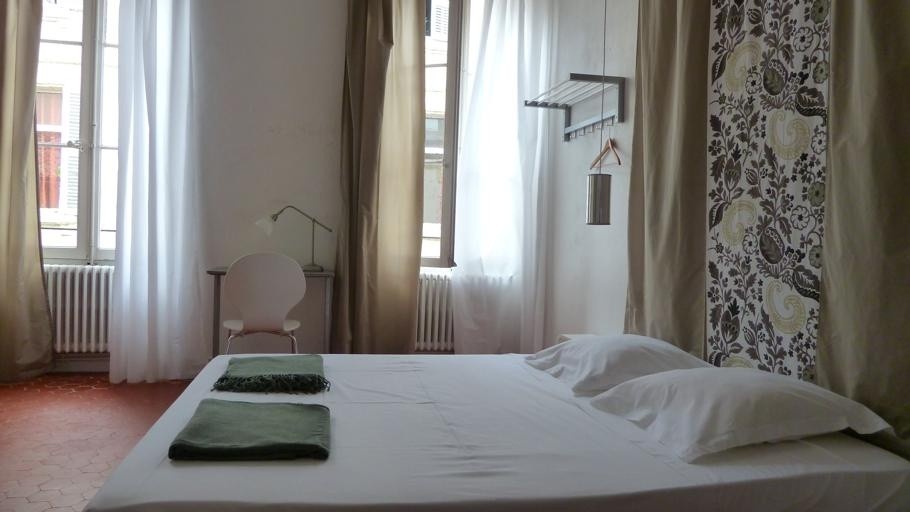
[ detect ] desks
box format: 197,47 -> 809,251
207,267 -> 336,355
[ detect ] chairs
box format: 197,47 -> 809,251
223,252 -> 307,353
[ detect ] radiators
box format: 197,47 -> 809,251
38,265 -> 116,354
407,273 -> 515,354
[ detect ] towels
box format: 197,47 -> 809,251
168,398 -> 332,460
212,357 -> 330,393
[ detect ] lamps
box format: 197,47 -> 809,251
587,1 -> 611,225
244,205 -> 331,272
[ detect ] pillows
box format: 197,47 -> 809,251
571,367 -> 896,461
524,334 -> 719,398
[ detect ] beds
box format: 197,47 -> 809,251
86,331 -> 909,509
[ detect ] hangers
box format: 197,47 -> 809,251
590,127 -> 621,168
